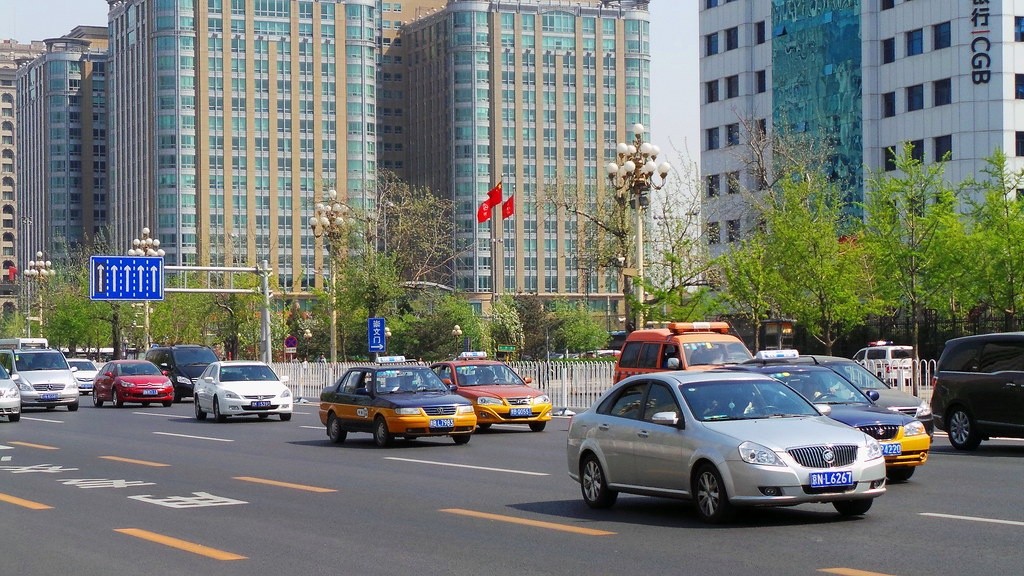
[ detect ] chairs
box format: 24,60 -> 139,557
367,375 -> 415,393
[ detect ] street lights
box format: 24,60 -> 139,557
303,329 -> 312,363
383,327 -> 391,357
128,228 -> 165,355
606,124 -> 672,332
452,324 -> 462,356
22,250 -> 56,338
308,190 -> 356,366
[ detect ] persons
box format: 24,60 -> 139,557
315,352 -> 327,363
800,375 -> 836,402
397,373 -> 419,391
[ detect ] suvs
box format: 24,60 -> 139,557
145,344 -> 225,403
931,331 -> 1024,451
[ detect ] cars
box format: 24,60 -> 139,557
551,348 -> 622,359
0,362 -> 22,422
319,356 -> 477,448
193,359 -> 293,424
712,349 -> 932,485
428,351 -> 553,432
91,360 -> 175,409
566,371 -> 886,528
66,358 -> 99,396
787,355 -> 934,445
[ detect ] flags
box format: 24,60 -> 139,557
478,199 -> 492,224
502,195 -> 514,220
487,181 -> 502,206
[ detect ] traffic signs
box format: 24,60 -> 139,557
368,317 -> 386,353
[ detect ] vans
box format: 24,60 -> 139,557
613,322 -> 754,385
0,349 -> 79,411
851,339 -> 920,386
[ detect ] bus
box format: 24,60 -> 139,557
0,337 -> 48,351
